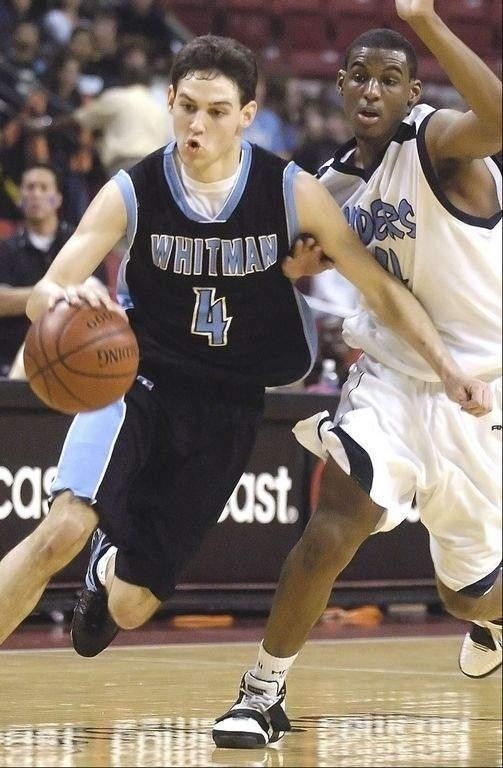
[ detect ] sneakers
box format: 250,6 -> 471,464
71,527 -> 119,657
459,619 -> 502,678
212,671 -> 291,748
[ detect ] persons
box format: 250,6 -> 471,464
1,34 -> 494,660
0,0 -> 499,383
209,1 -> 501,749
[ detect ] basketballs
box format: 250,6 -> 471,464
24,301 -> 139,415
